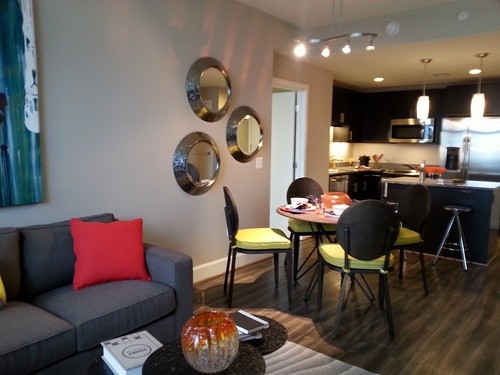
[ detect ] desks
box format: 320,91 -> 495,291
91,320 -> 378,375
277,200 -> 368,302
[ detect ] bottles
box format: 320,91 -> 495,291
419,164 -> 426,184
180,310 -> 239,374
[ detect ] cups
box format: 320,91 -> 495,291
315,197 -> 325,214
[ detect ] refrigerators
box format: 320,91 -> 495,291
439,116 -> 500,182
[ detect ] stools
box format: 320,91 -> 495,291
433,206 -> 472,270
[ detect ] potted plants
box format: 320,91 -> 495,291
359,155 -> 370,167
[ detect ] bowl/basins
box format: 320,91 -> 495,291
322,192 -> 351,211
331,205 -> 350,216
289,198 -> 309,208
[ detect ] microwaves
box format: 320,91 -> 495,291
388,118 -> 435,144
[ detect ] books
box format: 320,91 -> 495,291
229,310 -> 269,342
99,330 -> 163,375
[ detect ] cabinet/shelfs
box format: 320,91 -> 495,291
331,79 -> 500,200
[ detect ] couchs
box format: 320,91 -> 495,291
0,212 -> 194,375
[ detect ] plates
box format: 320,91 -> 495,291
284,203 -> 316,211
324,210 -> 341,218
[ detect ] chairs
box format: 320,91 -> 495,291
222,186 -> 293,307
284,177 -> 337,272
392,184 -> 431,297
319,200 -> 400,341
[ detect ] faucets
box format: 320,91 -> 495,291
329,155 -> 343,164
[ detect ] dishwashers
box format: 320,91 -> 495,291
330,175 -> 349,193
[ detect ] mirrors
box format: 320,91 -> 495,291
173,132 -> 220,195
186,57 -> 231,122
226,106 -> 263,163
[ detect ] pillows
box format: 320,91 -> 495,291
70,217 -> 151,290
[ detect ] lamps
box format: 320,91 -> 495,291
366,38 -> 376,51
321,45 -> 330,57
342,37 -> 352,54
416,59 -> 432,119
470,52 -> 489,117
